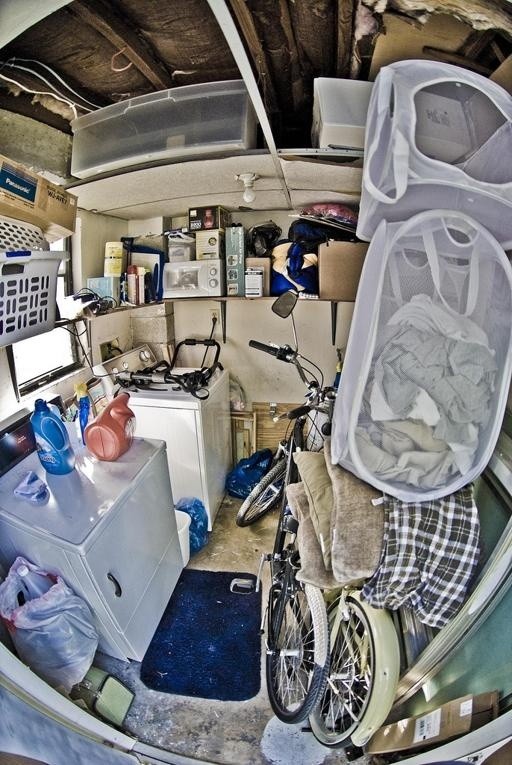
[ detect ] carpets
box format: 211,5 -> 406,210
139,566 -> 263,701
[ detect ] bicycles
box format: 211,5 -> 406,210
230,286 -> 328,726
273,386 -> 400,749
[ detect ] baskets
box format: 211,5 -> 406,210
0,215 -> 71,349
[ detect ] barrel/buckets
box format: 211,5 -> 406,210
83,392 -> 143,462
15,565 -> 54,604
30,399 -> 76,475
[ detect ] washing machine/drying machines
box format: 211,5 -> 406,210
92,344 -> 233,532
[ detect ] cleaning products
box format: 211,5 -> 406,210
73,381 -> 94,449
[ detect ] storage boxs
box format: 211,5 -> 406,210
309,77 -> 472,165
70,666 -> 136,729
86,275 -> 120,309
317,239 -> 369,301
103,241 -> 127,277
69,79 -> 252,180
0,152 -> 78,242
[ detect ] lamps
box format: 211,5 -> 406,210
234,173 -> 261,202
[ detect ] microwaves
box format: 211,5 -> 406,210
163,259 -> 226,299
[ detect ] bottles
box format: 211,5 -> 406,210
122,266 -> 156,306
19,566 -> 56,601
203,209 -> 215,227
280,502 -> 293,535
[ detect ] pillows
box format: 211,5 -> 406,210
284,439 -> 383,591
